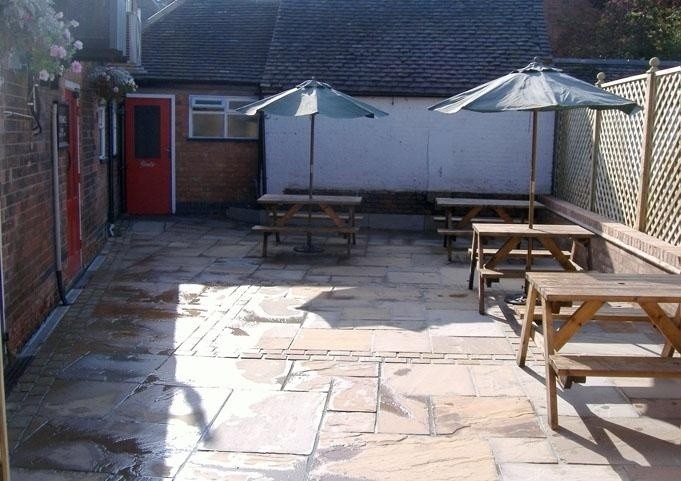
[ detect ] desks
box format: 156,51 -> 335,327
525,272 -> 681,302
472,223 -> 597,239
435,197 -> 546,210
257,194 -> 363,253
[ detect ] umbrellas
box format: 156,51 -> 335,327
233,75 -> 389,246
426,56 -> 642,295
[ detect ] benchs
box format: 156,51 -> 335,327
438,229 -> 473,260
516,306 -> 676,366
468,248 -> 571,289
478,269 -> 593,314
252,225 -> 360,260
269,212 -> 365,245
433,217 -> 535,247
545,355 -> 681,430
